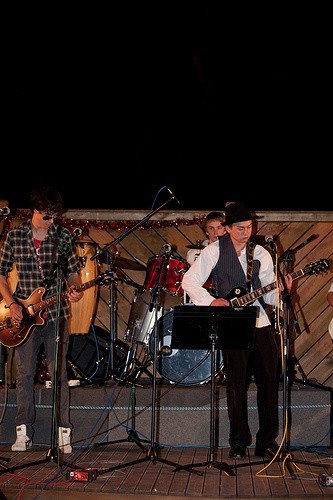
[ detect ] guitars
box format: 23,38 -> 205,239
0,269 -> 126,348
222,258 -> 330,307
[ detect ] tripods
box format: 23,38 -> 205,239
0,197 -> 330,484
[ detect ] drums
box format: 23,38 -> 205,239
123,288 -> 184,349
148,310 -> 224,386
64,240 -> 101,335
142,255 -> 192,296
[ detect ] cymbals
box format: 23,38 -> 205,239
97,253 -> 148,272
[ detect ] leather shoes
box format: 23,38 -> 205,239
229,446 -> 247,460
255,445 -> 287,461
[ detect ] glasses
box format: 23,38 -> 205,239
39,212 -> 59,222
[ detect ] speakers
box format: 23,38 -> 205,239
65,325 -> 130,384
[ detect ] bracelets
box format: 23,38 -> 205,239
9,302 -> 17,309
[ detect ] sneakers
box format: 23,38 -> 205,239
56,427 -> 72,454
11,425 -> 33,451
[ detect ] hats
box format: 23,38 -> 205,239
221,203 -> 258,227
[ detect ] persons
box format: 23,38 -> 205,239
0,192 -> 84,453
186,211 -> 228,268
0,197 -> 10,249
326,281 -> 333,450
181,203 -> 292,463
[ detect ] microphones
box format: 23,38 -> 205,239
161,244 -> 171,252
166,187 -> 180,204
265,234 -> 277,252
0,207 -> 10,215
159,345 -> 172,356
52,216 -> 62,235
